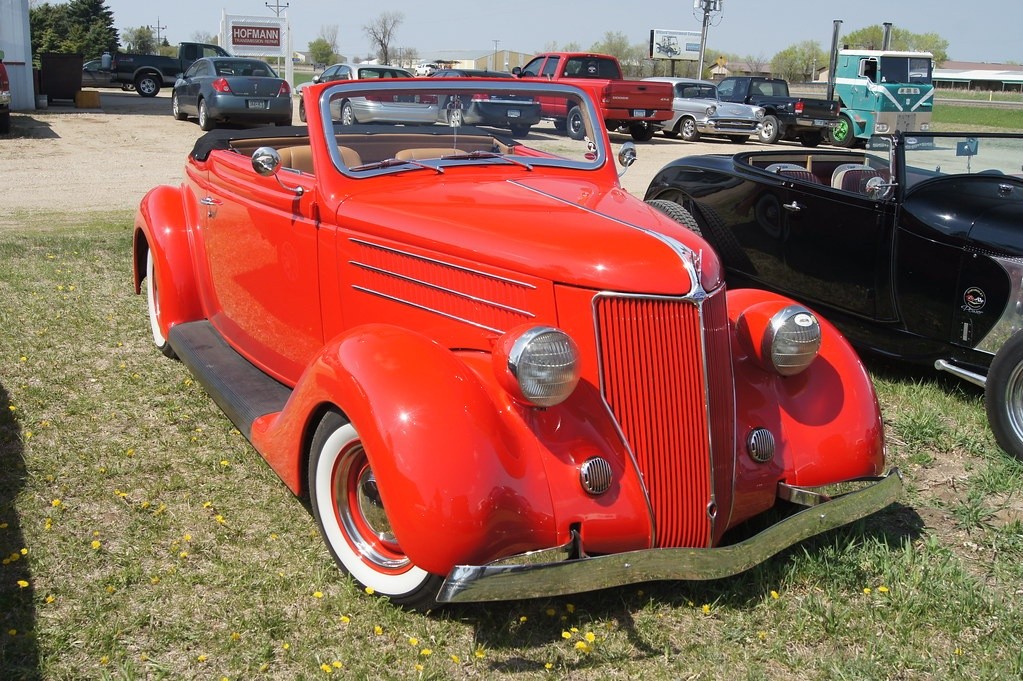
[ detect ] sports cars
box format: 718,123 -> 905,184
134,78 -> 903,604
642,148 -> 1023,461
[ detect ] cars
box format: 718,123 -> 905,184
297,63 -> 439,126
172,57 -> 293,130
430,70 -> 541,137
618,77 -> 760,140
82,59 -> 136,91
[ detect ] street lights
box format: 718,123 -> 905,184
693,0 -> 723,78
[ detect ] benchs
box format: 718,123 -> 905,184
219,69 -> 268,76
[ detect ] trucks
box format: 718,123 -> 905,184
720,20 -> 935,147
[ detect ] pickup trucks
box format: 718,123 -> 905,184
511,52 -> 676,140
112,42 -> 232,97
717,77 -> 840,147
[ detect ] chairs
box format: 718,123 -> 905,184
275,144 -> 361,174
397,147 -> 470,162
831,162 -> 886,193
764,162 -> 819,181
